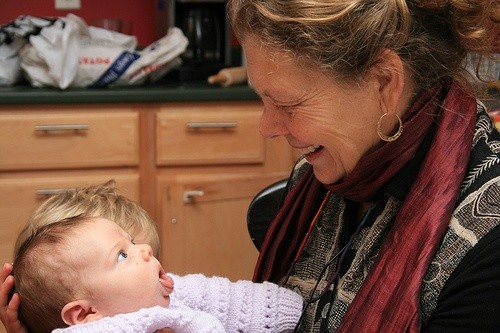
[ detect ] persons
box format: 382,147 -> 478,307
1,0 -> 500,333
7,178 -> 306,333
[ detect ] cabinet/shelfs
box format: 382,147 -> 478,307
151,105 -> 285,283
0,112 -> 142,333
